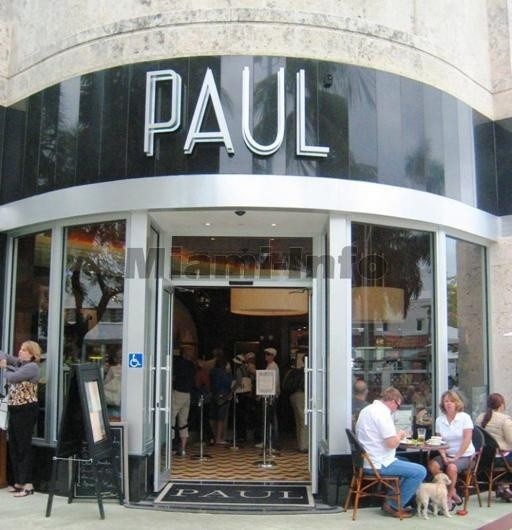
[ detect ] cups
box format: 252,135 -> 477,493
429,436 -> 442,445
417,428 -> 426,443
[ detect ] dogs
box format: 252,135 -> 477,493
415,472 -> 453,518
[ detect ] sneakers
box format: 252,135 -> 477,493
382,502 -> 414,518
496,487 -> 512,503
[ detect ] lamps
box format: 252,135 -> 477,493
352,287 -> 404,323
231,288 -> 308,316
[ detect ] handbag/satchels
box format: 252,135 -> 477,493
0,392 -> 12,431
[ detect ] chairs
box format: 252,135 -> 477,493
475,425 -> 512,507
458,427 -> 485,511
345,429 -> 402,521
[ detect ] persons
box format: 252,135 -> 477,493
475,394 -> 511,502
355,386 -> 427,518
100,349 -> 121,418
427,389 -> 476,515
353,380 -> 370,415
171,345 -> 308,457
1,340 -> 42,497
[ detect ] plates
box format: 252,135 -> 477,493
400,440 -> 424,445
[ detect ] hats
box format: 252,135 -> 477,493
264,348 -> 277,357
233,355 -> 245,365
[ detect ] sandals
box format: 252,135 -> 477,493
8,481 -> 23,493
15,486 -> 35,498
